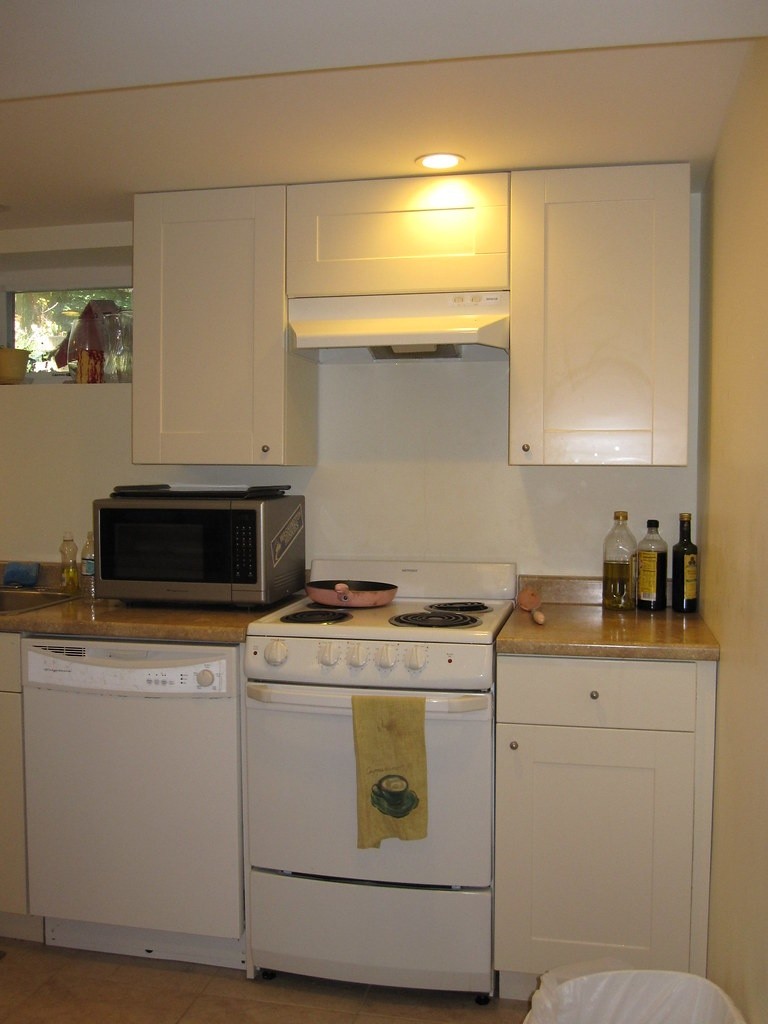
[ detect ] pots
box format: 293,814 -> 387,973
306,580 -> 397,605
0,345 -> 31,385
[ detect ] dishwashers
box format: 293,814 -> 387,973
20,637 -> 246,972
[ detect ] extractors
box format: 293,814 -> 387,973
289,291 -> 510,358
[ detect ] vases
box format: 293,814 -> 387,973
0,348 -> 31,381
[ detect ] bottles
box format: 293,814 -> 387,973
105,314 -> 132,382
81,532 -> 101,602
77,321 -> 103,384
603,512 -> 637,610
59,529 -> 78,593
672,513 -> 698,612
639,520 -> 667,611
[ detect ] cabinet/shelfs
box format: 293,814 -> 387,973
493,652 -> 716,1002
286,171 -> 510,299
131,183 -> 319,468
506,162 -> 692,467
0,630 -> 44,946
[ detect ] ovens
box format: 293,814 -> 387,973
247,679 -> 494,1003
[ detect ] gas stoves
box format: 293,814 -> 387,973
244,560 -> 518,689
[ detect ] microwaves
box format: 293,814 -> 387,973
93,485 -> 305,606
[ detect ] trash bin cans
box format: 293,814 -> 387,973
521,969 -> 747,1024
